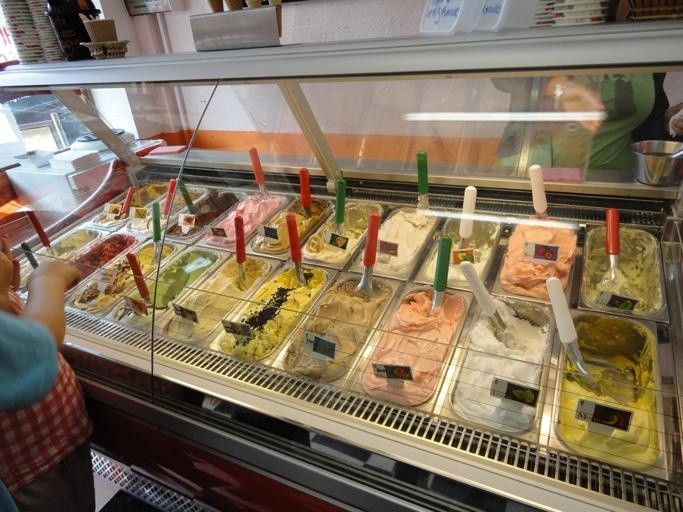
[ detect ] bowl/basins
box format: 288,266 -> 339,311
0,0 -> 64,65
628,140 -> 683,188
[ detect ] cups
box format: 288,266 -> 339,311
82,19 -> 120,42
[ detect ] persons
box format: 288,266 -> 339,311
491,72 -> 683,181
0,230 -> 94,511
